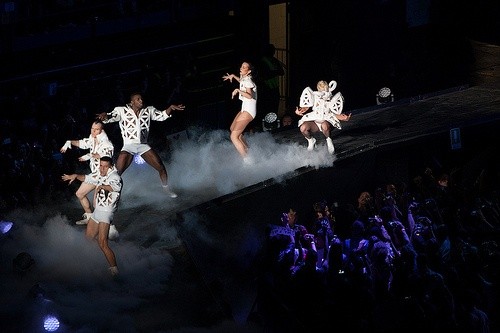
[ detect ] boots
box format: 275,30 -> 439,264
76,213 -> 93,224
109,225 -> 119,239
161,185 -> 177,198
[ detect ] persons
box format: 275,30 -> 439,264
59,92 -> 186,241
281,186 -> 500,333
86,156 -> 122,276
222,60 -> 258,157
284,80 -> 351,155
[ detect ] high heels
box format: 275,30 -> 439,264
305,136 -> 317,152
326,137 -> 335,155
